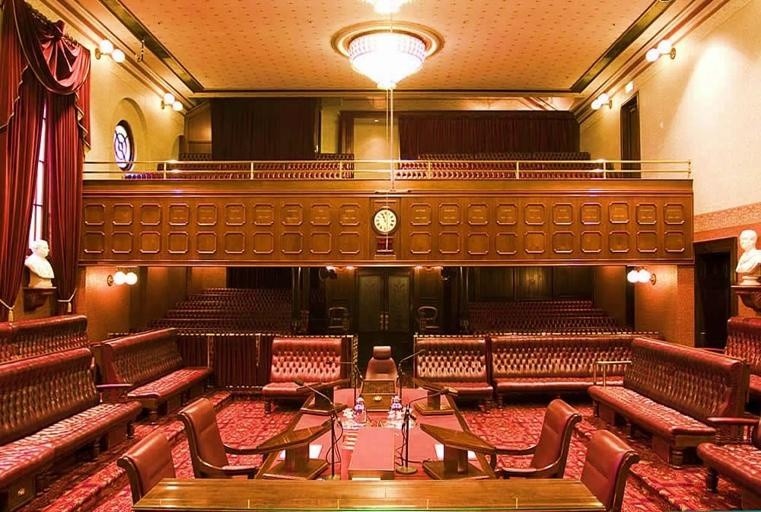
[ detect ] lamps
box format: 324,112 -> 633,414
645,39 -> 677,61
106,270 -> 139,286
627,267 -> 657,285
95,39 -> 125,64
591,92 -> 613,110
329,20 -> 447,91
160,93 -> 183,112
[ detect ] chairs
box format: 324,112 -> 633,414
490,398 -> 583,478
365,345 -> 400,387
122,151 -> 356,181
582,428 -> 641,511
177,397 -> 269,479
393,152 -> 625,180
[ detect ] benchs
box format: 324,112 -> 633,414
462,294 -> 634,335
413,332 -> 666,410
591,316 -> 761,510
131,285 -> 326,333
263,337 -> 352,409
0,312 -> 210,512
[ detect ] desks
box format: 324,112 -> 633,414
256,386 -> 498,480
132,477 -> 609,511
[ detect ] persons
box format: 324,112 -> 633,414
23,239 -> 55,287
734,228 -> 761,285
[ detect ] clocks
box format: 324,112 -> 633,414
372,207 -> 401,236
